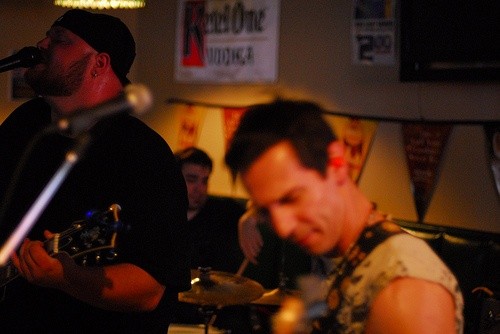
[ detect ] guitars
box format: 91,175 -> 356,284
0,203 -> 122,287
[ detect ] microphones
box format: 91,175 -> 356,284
56,84 -> 151,131
0,46 -> 43,74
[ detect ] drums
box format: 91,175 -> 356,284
167,324 -> 232,334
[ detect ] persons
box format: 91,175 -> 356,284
0,10 -> 191,334
225,100 -> 465,334
174,147 -> 327,289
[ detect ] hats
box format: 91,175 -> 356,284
54,9 -> 136,86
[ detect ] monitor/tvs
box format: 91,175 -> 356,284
395,0 -> 500,83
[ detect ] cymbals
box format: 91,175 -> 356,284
249,288 -> 293,305
178,269 -> 265,305
401,228 -> 482,247
